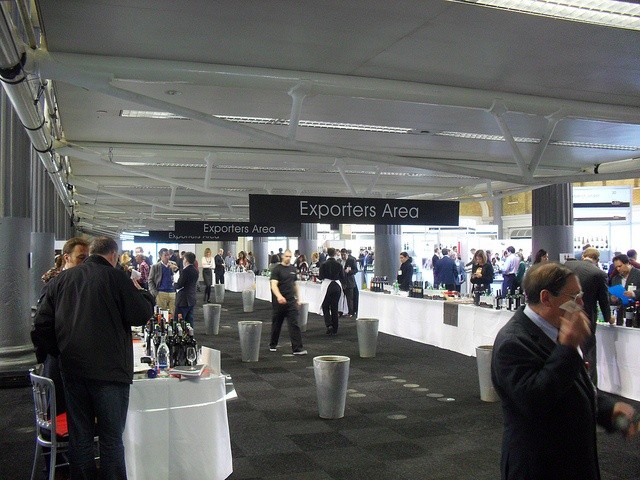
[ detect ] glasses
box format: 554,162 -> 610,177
549,290 -> 584,306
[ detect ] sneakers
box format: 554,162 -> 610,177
270,346 -> 277,353
292,349 -> 307,355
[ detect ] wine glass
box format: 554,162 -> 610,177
186,346 -> 197,371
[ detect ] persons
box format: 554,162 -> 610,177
117,245 -> 150,287
564,247 -> 616,388
270,248 -> 308,355
450,251 -> 466,292
298,254 -> 308,271
225,250 -> 236,269
470,249 -> 495,295
490,259 -> 640,480
434,248 -> 458,292
309,252 -> 320,272
214,248 -> 226,284
236,251 -> 248,269
29,238 -> 89,480
453,246 -> 461,260
397,252 -> 414,292
514,251 -> 526,295
486,250 -> 508,272
499,246 -> 520,296
248,252 -> 255,266
172,251 -> 199,327
293,250 -> 301,266
348,249 -> 356,260
201,247 -> 216,303
276,247 -> 284,263
337,249 -> 359,318
359,246 -> 374,271
30,235 -> 156,480
180,251 -> 185,261
318,247 -> 344,335
168,249 -> 183,269
269,250 -> 274,256
610,249 -> 640,278
335,248 -> 341,260
432,248 -> 441,290
534,249 -> 549,264
516,248 -> 524,262
609,254 -> 640,310
147,249 -> 180,316
319,247 -> 327,262
466,248 -> 477,273
608,251 -> 622,276
194,260 -> 200,293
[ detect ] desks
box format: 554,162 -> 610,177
213,268 -> 255,291
591,318 -> 640,404
356,284 -> 518,356
255,275 -> 347,316
124,320 -> 235,480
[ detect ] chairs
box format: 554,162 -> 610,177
28,371 -> 101,476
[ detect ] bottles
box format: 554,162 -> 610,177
625,298 -> 633,327
632,301 -> 640,329
371,274 -> 390,292
495,290 -> 500,310
409,280 -> 423,297
156,305 -> 171,331
616,297 -> 624,327
156,332 -> 171,373
171,314 -> 187,367
143,305 -> 156,365
505,286 -> 525,311
186,327 -> 198,366
498,289 -> 503,309
394,279 -> 399,294
474,284 -> 494,307
